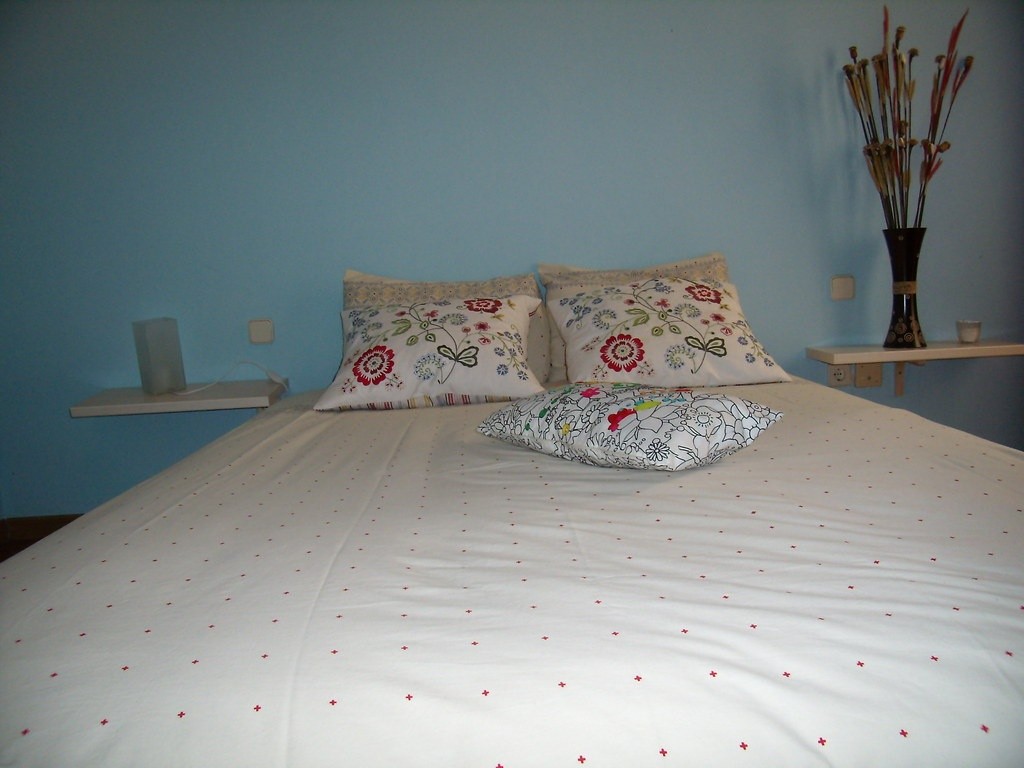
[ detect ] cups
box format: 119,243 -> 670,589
956,320 -> 982,342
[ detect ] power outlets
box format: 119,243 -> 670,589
828,364 -> 852,387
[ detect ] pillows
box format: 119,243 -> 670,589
546,278 -> 792,386
538,253 -> 730,383
343,269 -> 550,384
313,295 -> 546,411
476,381 -> 784,469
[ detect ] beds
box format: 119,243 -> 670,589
0,373 -> 1024,768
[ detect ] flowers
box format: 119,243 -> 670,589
842,5 -> 974,228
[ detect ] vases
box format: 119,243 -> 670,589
882,228 -> 928,348
